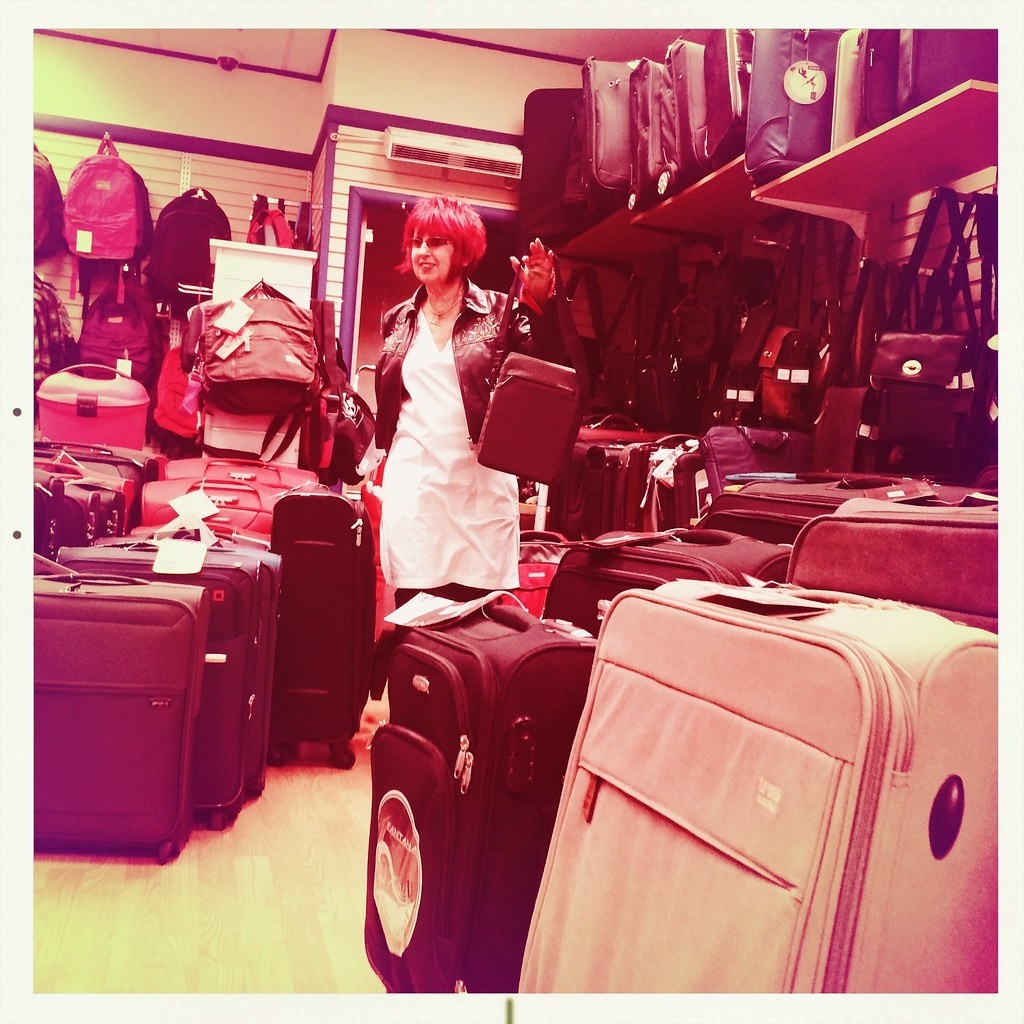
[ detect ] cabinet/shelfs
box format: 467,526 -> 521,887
202,238 -> 318,468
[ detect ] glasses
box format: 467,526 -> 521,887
407,236 -> 453,249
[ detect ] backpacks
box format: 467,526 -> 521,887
34,134 -> 382,485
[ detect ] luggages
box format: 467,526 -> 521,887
32,431 -> 997,994
516,29 -> 998,256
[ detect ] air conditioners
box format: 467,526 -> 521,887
384,126 -> 523,179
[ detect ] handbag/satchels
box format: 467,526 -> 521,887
475,184 -> 999,480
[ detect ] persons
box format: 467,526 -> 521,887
375,196 -> 555,609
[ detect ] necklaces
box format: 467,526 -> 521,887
427,296 -> 461,326
